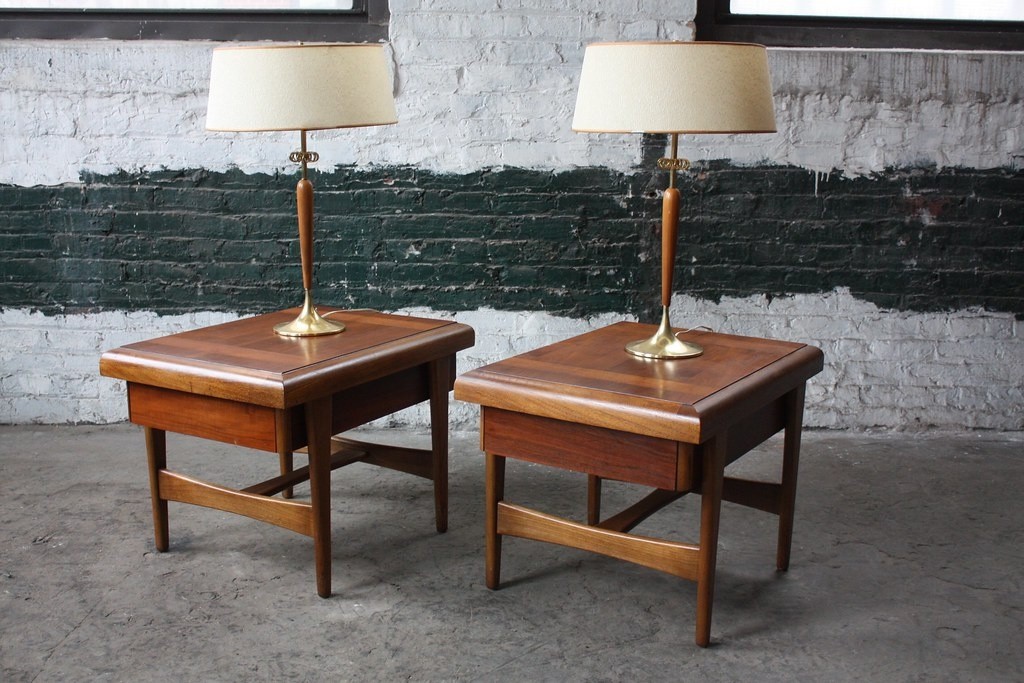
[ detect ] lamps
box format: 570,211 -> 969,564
572,42 -> 777,360
207,41 -> 398,336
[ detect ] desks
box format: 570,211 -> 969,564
100,305 -> 476,598
454,323 -> 823,646
